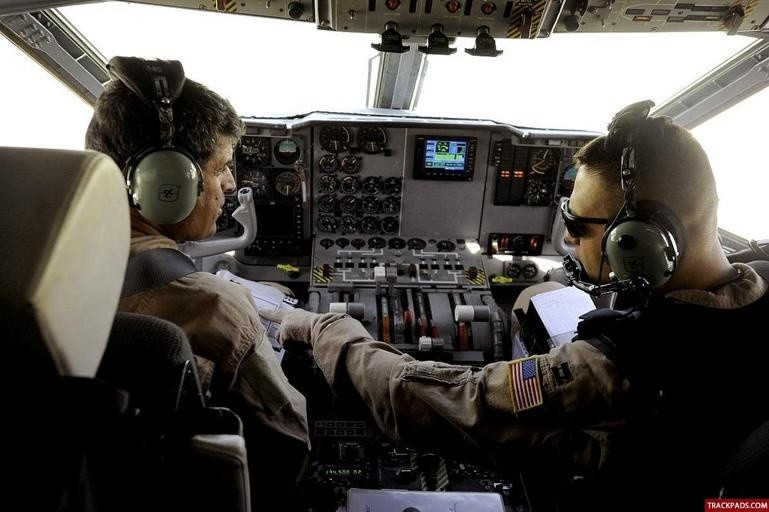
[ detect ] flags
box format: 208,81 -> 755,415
508,355 -> 543,414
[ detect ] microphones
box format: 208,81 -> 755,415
562,253 -> 649,297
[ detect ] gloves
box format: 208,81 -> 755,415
258,307 -> 347,347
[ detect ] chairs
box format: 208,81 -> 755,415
0,150 -> 251,511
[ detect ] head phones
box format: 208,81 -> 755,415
106,56 -> 204,225
603,100 -> 686,293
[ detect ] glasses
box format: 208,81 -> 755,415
559,195 -> 615,240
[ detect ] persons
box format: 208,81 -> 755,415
258,115 -> 769,512
83,75 -> 312,512
